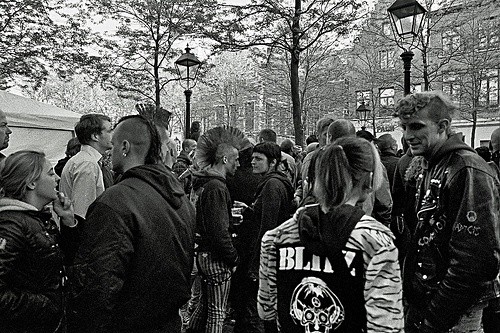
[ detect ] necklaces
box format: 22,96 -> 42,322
211,169 -> 224,177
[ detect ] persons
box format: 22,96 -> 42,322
395,92 -> 500,333
475,129 -> 500,333
134,103 -> 302,333
70,115 -> 197,333
0,112 -> 113,333
257,136 -> 406,333
294,119 -> 419,277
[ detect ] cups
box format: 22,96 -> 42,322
232,207 -> 241,227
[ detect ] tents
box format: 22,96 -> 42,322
0,90 -> 83,165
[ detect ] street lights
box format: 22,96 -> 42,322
355,94 -> 372,132
386,0 -> 426,157
174,43 -> 204,140
